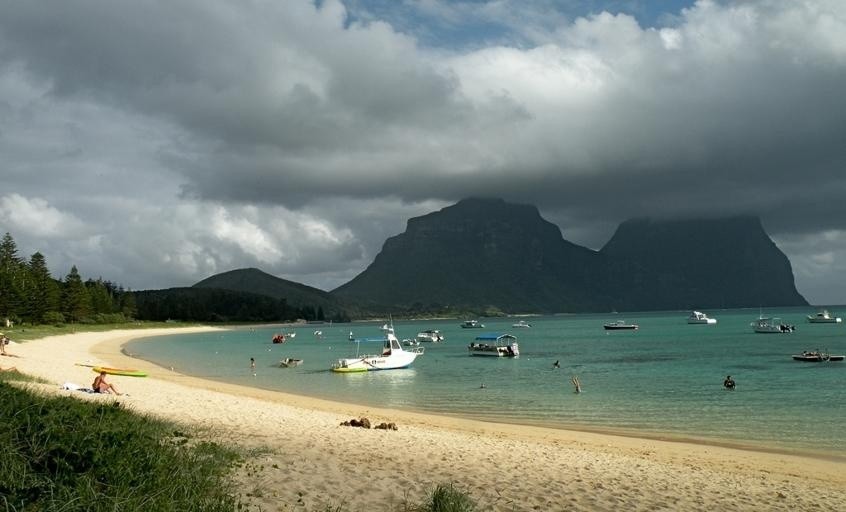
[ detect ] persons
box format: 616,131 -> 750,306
553,359 -> 561,368
92,371 -> 122,396
571,374 -> 583,392
250,358 -> 256,367
723,375 -> 736,392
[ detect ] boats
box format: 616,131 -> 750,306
604,321 -> 638,330
807,312 -> 842,323
750,317 -> 796,333
686,311 -> 716,324
333,335 -> 424,373
460,320 -> 485,328
792,354 -> 844,361
512,321 -> 532,328
417,329 -> 446,342
280,360 -> 301,367
467,335 -> 517,356
